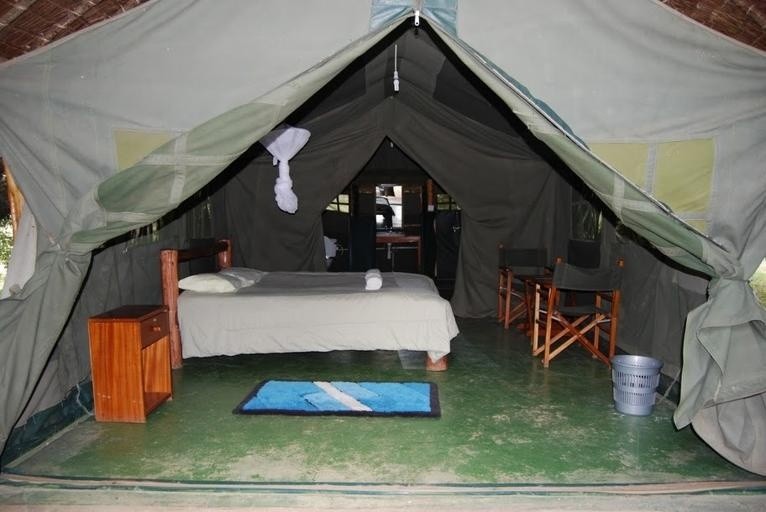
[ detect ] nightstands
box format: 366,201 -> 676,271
88,304 -> 173,423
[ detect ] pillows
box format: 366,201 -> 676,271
177,265 -> 268,294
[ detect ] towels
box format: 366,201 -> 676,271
364,267 -> 383,291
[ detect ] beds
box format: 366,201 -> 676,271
159,237 -> 449,372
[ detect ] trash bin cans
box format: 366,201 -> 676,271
611,355 -> 664,417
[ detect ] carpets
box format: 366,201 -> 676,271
232,378 -> 442,420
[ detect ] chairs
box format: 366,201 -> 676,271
497,245 -> 624,368
